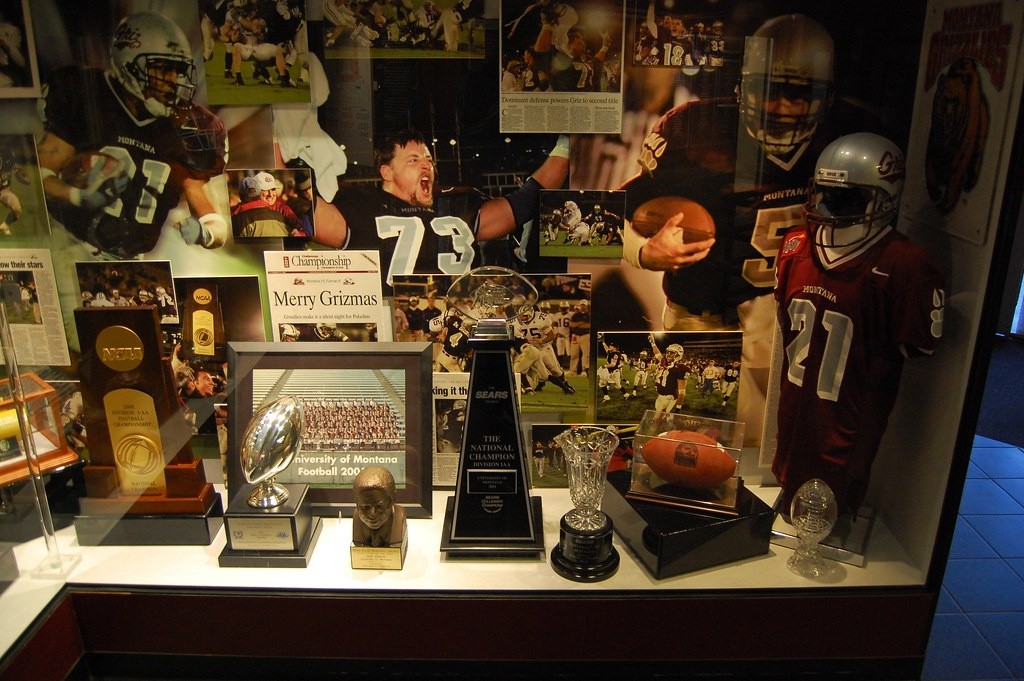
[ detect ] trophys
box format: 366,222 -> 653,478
74,307 -> 225,546
439,319 -> 546,559
786,478 -> 839,577
551,425 -> 621,583
219,394 -> 324,568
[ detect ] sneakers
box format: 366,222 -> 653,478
224,70 -> 296,87
0,221 -> 12,236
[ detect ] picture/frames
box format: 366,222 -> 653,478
227,341 -> 434,518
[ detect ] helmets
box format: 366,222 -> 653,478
803,132 -> 906,228
109,10 -> 195,118
560,301 -> 569,307
738,13 -> 833,154
640,351 -> 647,357
541,302 -> 550,308
511,294 -> 526,306
519,305 -> 534,322
666,344 -> 684,362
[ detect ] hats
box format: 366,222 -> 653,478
710,360 -> 715,363
409,296 -> 417,302
254,172 -> 277,191
580,299 -> 588,305
574,305 -> 580,309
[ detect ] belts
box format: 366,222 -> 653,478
442,350 -> 456,360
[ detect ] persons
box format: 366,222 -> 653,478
285,129 -> 571,295
0,272 -> 42,324
435,399 -> 467,453
36,10 -> 230,261
252,403 -> 406,452
80,270 -> 177,325
391,275 -> 592,397
230,170 -> 316,237
351,466 -> 406,547
0,143 -> 32,236
201,1 -> 729,92
596,331 -> 740,435
60,342 -> 230,489
543,198 -> 625,248
533,440 -> 568,478
752,131 -> 946,552
619,13 -> 840,333
278,322 -> 378,343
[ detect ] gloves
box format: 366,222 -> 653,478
180,217 -> 211,245
548,134 -> 577,158
77,155 -> 131,215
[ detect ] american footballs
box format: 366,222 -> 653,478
640,429 -> 737,489
55,151 -> 127,189
632,196 -> 716,245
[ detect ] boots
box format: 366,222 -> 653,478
511,371 -> 576,395
